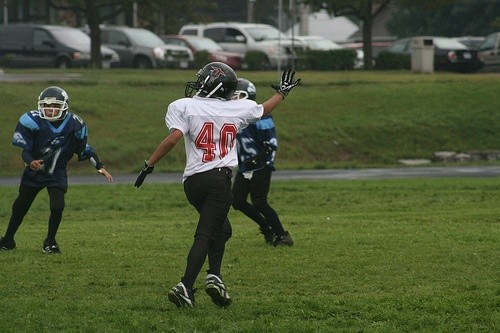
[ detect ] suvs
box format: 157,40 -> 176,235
0,25 -> 121,69
77,27 -> 195,71
178,20 -> 297,70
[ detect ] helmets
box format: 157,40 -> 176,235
270,68 -> 301,99
37,87 -> 70,121
229,78 -> 257,101
185,61 -> 237,100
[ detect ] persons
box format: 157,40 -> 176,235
229,78 -> 295,247
0,86 -> 113,255
134,62 -> 301,308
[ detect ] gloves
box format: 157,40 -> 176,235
134,160 -> 154,188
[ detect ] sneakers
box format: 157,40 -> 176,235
167,282 -> 197,308
42,238 -> 60,253
0,237 -> 16,252
204,274 -> 231,308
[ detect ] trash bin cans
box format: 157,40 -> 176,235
410,37 -> 434,73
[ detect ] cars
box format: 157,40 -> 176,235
160,34 -> 242,70
287,35 -> 500,72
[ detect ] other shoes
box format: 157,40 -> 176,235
260,227 -> 274,245
275,232 -> 294,246
